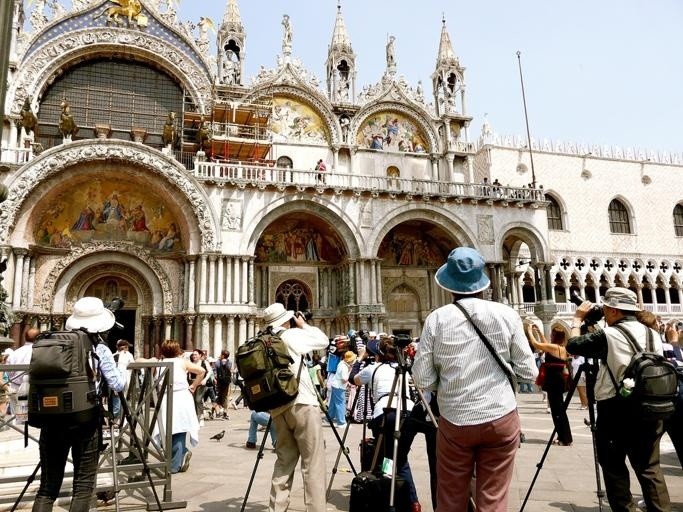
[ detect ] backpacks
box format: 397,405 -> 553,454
216,358 -> 232,384
231,369 -> 244,385
25,330 -> 109,428
234,328 -> 304,413
602,324 -> 676,420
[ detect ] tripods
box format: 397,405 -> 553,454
326,362 -> 378,503
368,348 -> 476,512
11,330 -> 165,512
241,367 -> 361,512
520,326 -> 629,512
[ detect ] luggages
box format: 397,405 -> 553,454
347,469 -> 411,512
358,436 -> 383,473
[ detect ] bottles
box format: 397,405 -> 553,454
15,374 -> 30,424
618,385 -> 635,397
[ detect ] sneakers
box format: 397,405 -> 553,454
637,498 -> 648,512
179,450 -> 191,472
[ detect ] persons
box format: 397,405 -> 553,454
319,159 -> 327,184
281,14 -> 293,44
36,193 -> 180,251
223,50 -> 239,85
315,161 -> 322,184
197,17 -> 217,41
481,178 -> 538,199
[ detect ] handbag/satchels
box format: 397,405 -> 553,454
206,378 -> 213,387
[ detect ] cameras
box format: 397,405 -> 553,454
289,311 -> 313,327
673,320 -> 683,332
391,329 -> 413,347
567,290 -> 604,325
358,328 -> 375,357
101,297 -> 125,330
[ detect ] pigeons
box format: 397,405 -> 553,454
200,406 -> 224,421
584,418 -> 591,427
97,489 -> 122,505
210,430 -> 226,442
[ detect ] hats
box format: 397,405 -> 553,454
262,302 -> 294,329
343,351 -> 357,362
116,339 -> 132,347
65,296 -> 116,333
366,340 -> 381,355
433,247 -> 490,295
597,286 -> 644,312
